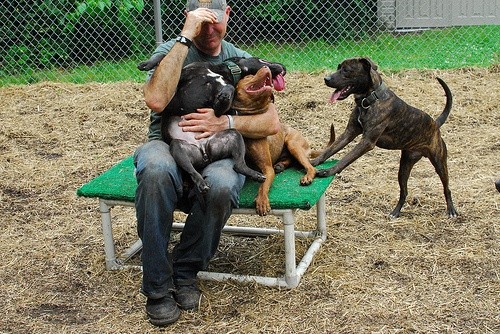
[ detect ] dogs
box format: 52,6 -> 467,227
309,58 -> 459,220
137,53 -> 336,216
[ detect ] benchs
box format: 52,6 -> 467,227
75,151 -> 339,288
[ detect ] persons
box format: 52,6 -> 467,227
134,0 -> 279,325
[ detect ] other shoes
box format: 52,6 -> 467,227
146,291 -> 181,325
171,284 -> 200,310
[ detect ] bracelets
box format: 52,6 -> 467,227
176,35 -> 192,49
227,115 -> 233,128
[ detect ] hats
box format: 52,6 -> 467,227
186,0 -> 226,22
